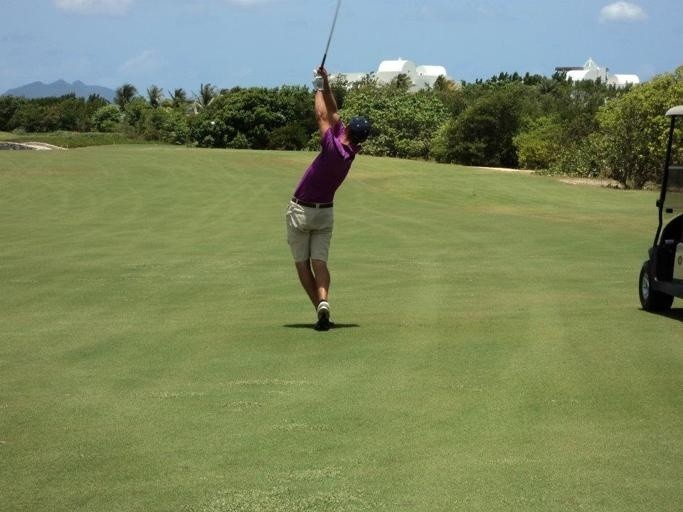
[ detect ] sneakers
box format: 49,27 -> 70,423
314,301 -> 330,331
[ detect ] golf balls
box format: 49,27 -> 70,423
211,121 -> 214,124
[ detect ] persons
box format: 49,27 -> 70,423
285,64 -> 371,331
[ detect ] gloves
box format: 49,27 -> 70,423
312,70 -> 325,91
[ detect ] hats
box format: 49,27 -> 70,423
350,116 -> 370,143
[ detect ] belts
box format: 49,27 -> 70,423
292,198 -> 334,208
